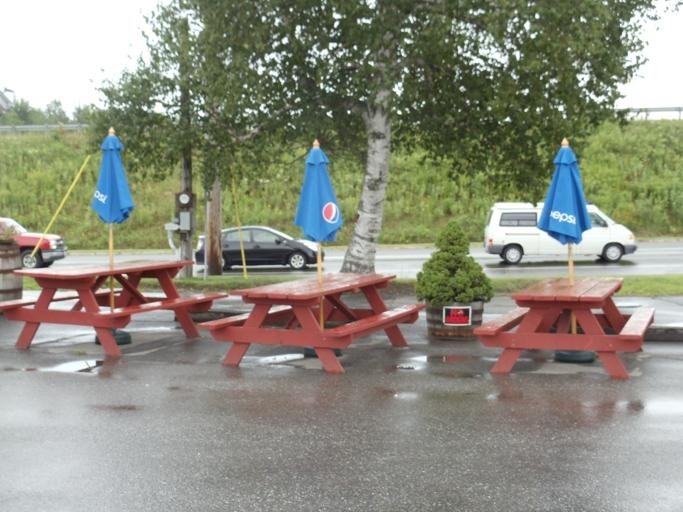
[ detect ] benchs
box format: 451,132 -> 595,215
473,307 -> 654,379
198,304 -> 425,372
0,289 -> 228,355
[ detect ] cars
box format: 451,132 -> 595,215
0,217 -> 68,268
194,225 -> 323,271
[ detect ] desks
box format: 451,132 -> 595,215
222,274 -> 407,373
490,278 -> 643,379
14,260 -> 198,356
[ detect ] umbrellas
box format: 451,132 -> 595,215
535,134 -> 593,340
292,137 -> 346,331
88,125 -> 135,313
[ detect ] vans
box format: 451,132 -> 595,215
484,200 -> 637,262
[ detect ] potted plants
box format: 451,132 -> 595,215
411,219 -> 492,343
0,223 -> 25,304
